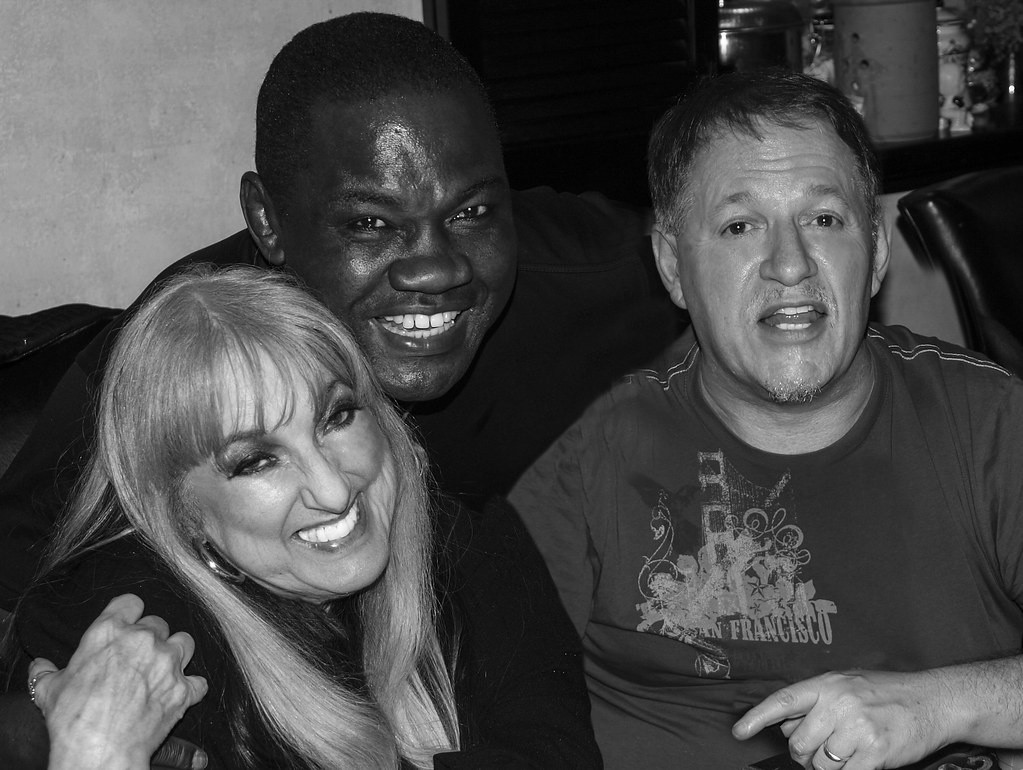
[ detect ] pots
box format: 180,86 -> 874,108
718,0 -> 805,74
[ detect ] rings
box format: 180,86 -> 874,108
20,669 -> 56,705
823,738 -> 853,766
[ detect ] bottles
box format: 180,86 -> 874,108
840,35 -> 876,139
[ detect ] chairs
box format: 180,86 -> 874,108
897,170 -> 1022,375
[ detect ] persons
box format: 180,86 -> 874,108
499,69 -> 1023,770
2,271 -> 605,770
103,8 -> 666,758
